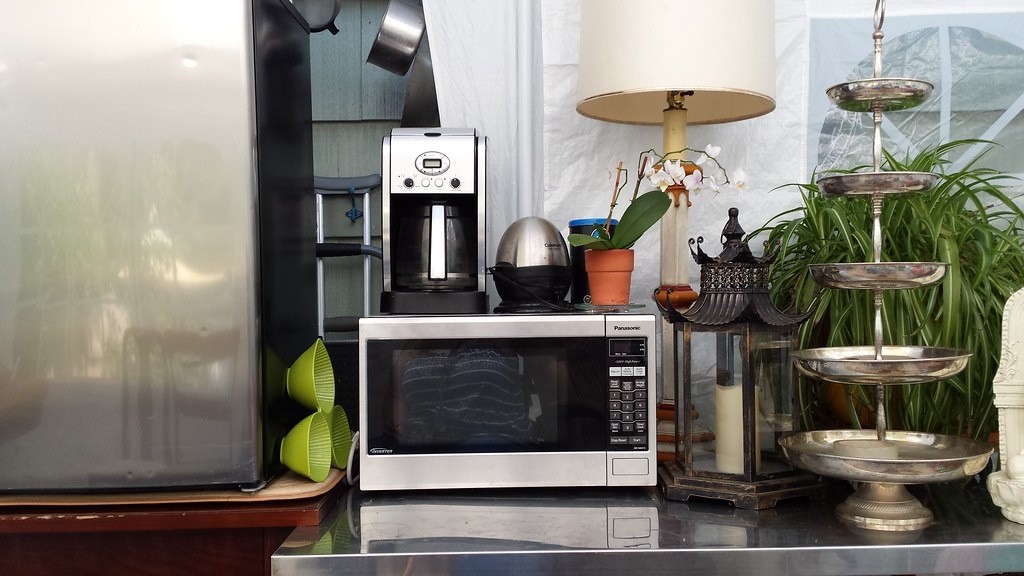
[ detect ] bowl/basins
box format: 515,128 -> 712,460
365,0 -> 427,77
279,411 -> 332,483
327,404 -> 352,470
287,337 -> 336,415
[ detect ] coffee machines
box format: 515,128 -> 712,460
380,127 -> 490,316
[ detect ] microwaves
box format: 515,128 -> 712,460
358,314 -> 658,491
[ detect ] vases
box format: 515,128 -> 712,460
584,248 -> 635,306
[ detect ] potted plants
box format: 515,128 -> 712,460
742,140 -> 1024,450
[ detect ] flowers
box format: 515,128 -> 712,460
567,143 -> 748,251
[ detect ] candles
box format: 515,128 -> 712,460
714,380 -> 762,476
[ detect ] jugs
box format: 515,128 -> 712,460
399,202 -> 472,291
